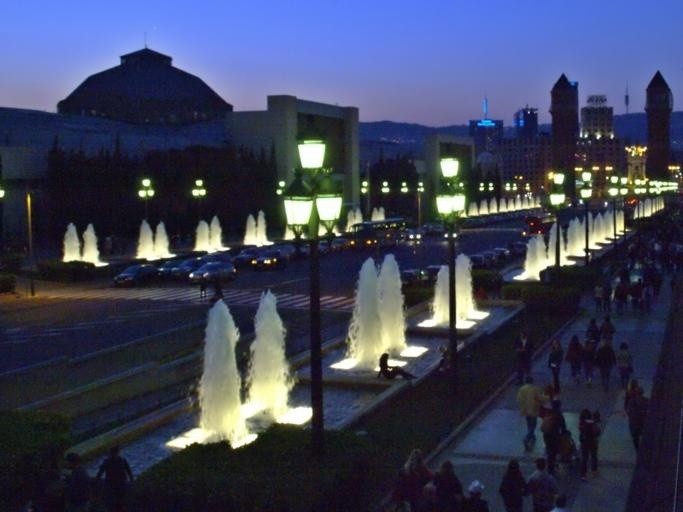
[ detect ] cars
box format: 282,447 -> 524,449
111,247 -> 255,289
416,235 -> 532,290
251,215 -> 464,271
463,207 -> 555,235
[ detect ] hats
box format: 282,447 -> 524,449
468,480 -> 485,494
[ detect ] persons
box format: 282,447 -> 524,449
96,444 -> 133,511
199,273 -> 209,303
389,205 -> 683,508
210,278 -> 224,309
377,353 -> 417,381
62,452 -> 92,512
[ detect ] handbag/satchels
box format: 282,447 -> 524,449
556,493 -> 567,507
629,363 -> 633,372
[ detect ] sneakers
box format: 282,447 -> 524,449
522,438 -> 530,448
580,476 -> 589,481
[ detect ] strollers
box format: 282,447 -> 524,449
559,430 -> 580,464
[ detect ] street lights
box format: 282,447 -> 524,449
548,169 -> 564,295
473,179 -> 534,209
576,167 -> 594,269
190,178 -> 207,225
605,173 -> 679,251
0,188 -> 6,218
358,178 -> 424,235
275,179 -> 286,197
278,132 -> 346,462
136,177 -> 156,227
21,188 -> 43,296
432,156 -> 469,402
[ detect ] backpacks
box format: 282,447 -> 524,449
540,413 -> 558,433
